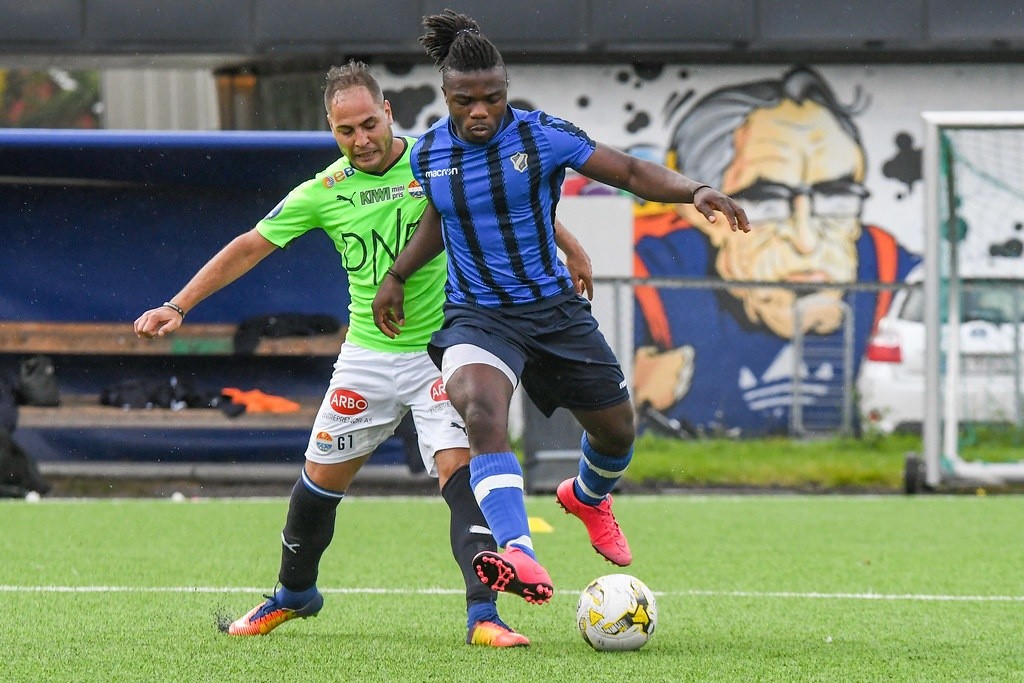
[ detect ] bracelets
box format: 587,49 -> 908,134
692,184 -> 712,198
385,267 -> 406,285
162,302 -> 186,320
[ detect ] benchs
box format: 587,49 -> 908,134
0,320 -> 351,356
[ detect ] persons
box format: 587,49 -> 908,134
374,8 -> 757,607
130,11 -> 533,646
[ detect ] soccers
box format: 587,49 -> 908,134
579,574 -> 658,653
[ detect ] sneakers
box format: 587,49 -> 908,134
474,548 -> 554,606
226,595 -> 322,637
466,620 -> 529,649
555,479 -> 632,565
